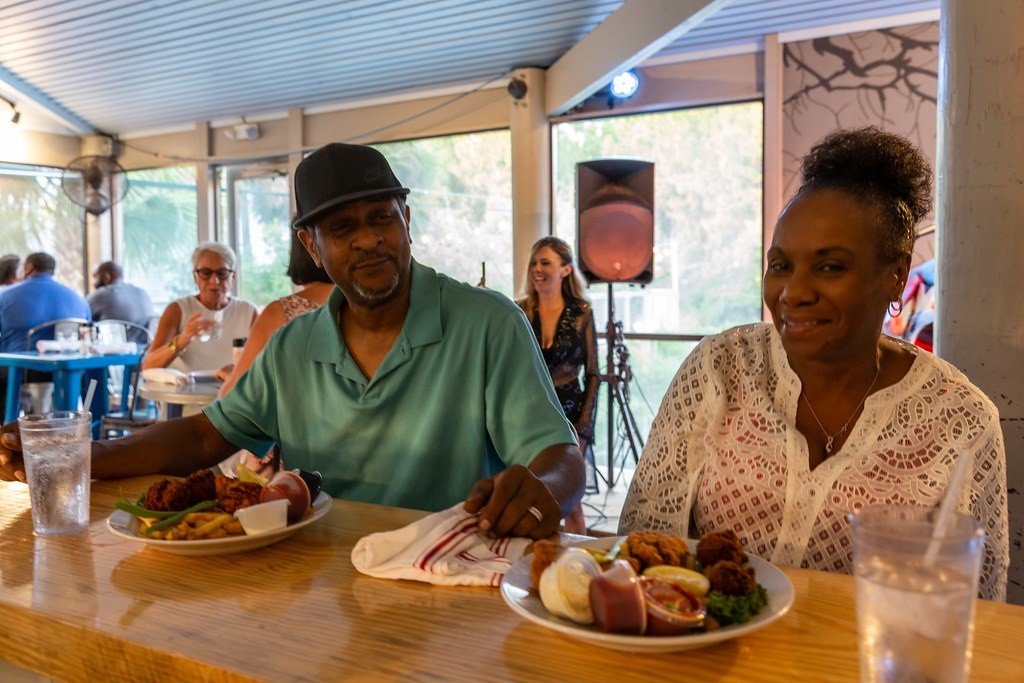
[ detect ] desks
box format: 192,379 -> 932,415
0,343 -> 146,440
137,373 -> 224,420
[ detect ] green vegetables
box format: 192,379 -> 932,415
706,582 -> 770,624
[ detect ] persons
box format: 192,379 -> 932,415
0,252 -> 92,353
514,236 -> 603,538
620,125 -> 1010,603
87,261 -> 155,327
0,142 -> 586,540
142,242 -> 257,373
218,215 -> 335,394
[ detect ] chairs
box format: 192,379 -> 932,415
20,318 -> 96,417
92,319 -> 153,397
99,345 -> 159,441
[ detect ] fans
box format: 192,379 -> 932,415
59,154 -> 131,218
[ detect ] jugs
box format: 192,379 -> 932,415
100,323 -> 127,353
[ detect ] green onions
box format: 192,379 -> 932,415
114,487 -> 219,533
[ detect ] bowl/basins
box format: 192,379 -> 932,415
643,562 -> 710,593
259,471 -> 311,519
233,499 -> 292,535
588,559 -> 648,633
638,576 -> 706,633
290,468 -> 323,503
539,546 -> 603,624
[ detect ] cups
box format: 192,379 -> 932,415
201,310 -> 223,343
852,507 -> 984,683
17,410 -> 92,535
57,323 -> 79,342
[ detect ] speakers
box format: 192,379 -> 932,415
575,159 -> 654,289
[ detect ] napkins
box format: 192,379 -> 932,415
350,499 -> 534,588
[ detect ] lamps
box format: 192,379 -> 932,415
593,69 -> 640,111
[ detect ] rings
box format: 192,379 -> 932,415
529,506 -> 542,522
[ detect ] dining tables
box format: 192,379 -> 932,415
0,475 -> 1024,683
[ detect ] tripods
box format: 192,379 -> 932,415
598,281 -> 645,488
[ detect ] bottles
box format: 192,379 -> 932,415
233,337 -> 247,365
81,322 -> 97,357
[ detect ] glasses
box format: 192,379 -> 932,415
195,267 -> 233,280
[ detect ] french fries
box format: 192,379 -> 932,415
138,512 -> 244,540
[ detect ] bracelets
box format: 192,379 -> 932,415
168,337 -> 186,355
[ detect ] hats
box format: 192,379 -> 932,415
294,143 -> 410,228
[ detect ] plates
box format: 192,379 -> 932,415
499,536 -> 795,652
190,370 -> 221,381
105,490 -> 333,556
